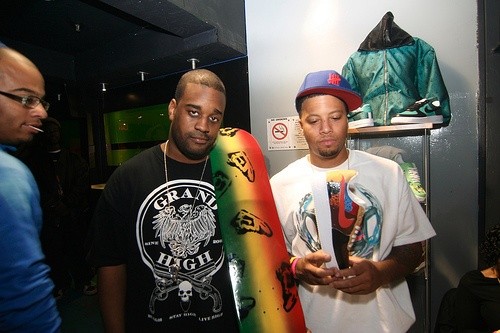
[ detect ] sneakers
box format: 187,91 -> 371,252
313,169 -> 367,281
392,152 -> 427,201
346,104 -> 375,129
390,98 -> 443,124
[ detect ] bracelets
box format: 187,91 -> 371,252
290,257 -> 300,277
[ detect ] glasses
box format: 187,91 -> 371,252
0,91 -> 50,111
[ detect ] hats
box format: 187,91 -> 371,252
296,70 -> 362,111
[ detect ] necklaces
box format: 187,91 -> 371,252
164,140 -> 209,281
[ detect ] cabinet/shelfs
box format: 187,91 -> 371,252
346,123 -> 434,333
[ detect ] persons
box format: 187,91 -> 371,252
85,69 -> 241,333
27,117 -> 98,300
0,48 -> 62,333
270,70 -> 436,333
